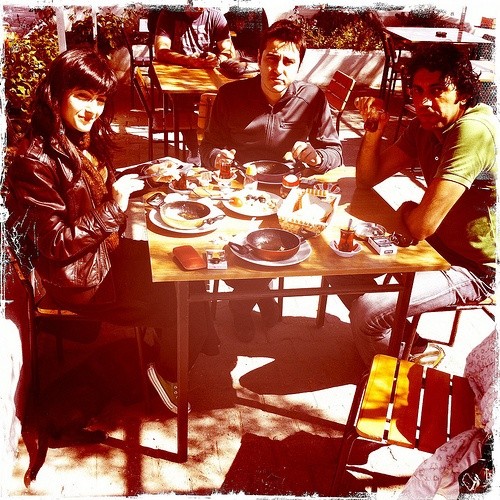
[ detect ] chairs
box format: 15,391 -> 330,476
120,10 -> 217,160
325,31 -> 411,136
332,273 -> 499,497
5,232 -> 152,400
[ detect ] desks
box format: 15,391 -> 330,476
387,27 -> 494,52
148,61 -> 252,157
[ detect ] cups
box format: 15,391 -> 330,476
174,169 -> 187,191
219,159 -> 231,179
278,173 -> 303,198
338,225 -> 355,251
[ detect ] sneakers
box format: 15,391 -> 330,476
146,365 -> 192,415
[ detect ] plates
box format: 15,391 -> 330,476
149,161 -> 312,266
314,184 -> 340,195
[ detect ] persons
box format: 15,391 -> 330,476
9,47 -> 206,412
157,0 -> 238,162
201,21 -> 344,342
328,37 -> 499,383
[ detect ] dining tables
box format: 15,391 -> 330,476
142,167 -> 449,463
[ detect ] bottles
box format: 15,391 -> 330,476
243,165 -> 257,189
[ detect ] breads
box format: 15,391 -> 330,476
287,193 -> 333,225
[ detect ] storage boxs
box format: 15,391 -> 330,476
368,236 -> 397,255
206,250 -> 228,269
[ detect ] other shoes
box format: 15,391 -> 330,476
256,297 -> 279,323
353,345 -> 446,385
230,298 -> 254,343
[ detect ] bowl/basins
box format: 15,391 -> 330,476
328,237 -> 364,258
248,161 -> 291,182
159,201 -> 210,229
245,229 -> 301,261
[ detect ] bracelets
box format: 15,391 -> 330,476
216,53 -> 220,61
399,202 -> 417,221
317,151 -> 323,162
